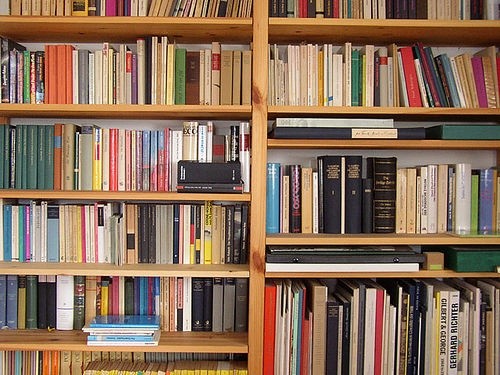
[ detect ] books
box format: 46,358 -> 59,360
1,1 -> 499,374
